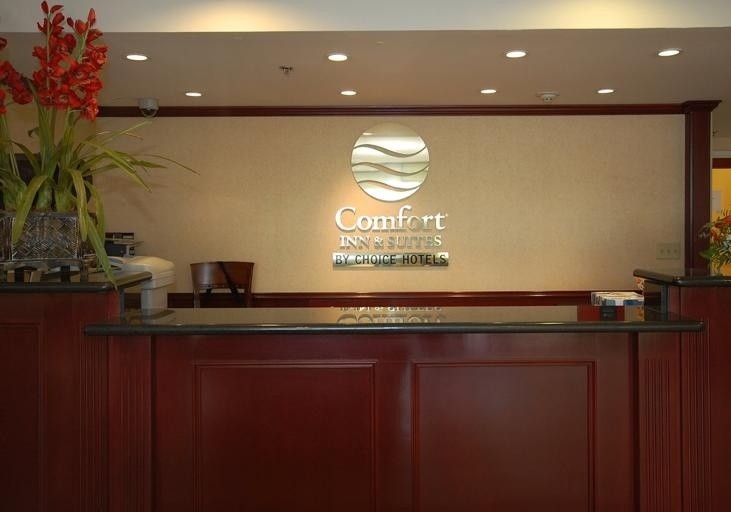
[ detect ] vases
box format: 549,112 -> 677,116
0,210 -> 96,282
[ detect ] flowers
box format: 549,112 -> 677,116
0,0 -> 199,288
697,210 -> 731,273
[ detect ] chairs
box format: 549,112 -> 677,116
189,261 -> 255,309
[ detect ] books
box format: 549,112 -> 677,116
590,291 -> 644,306
